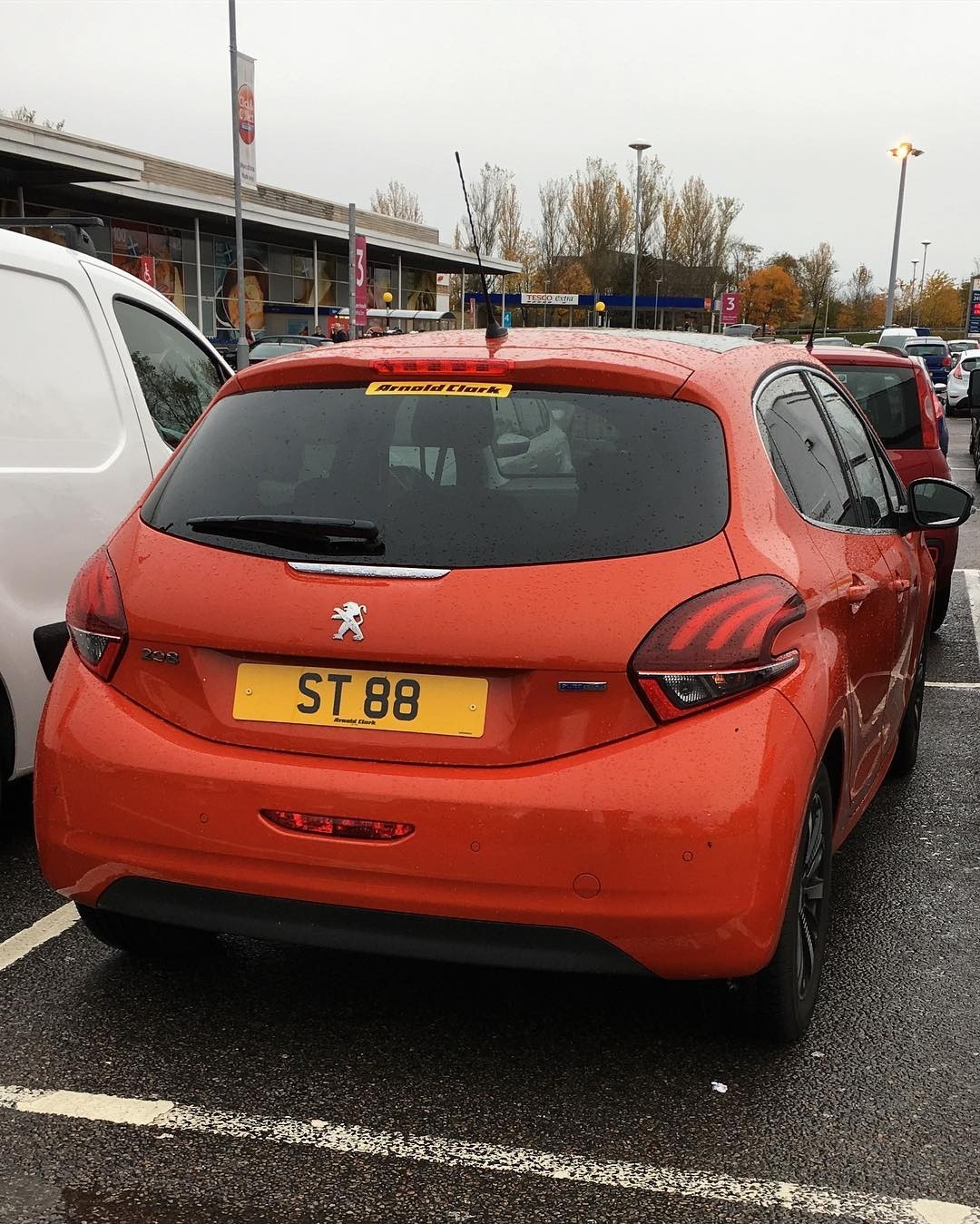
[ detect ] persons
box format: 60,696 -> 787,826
311,326 -> 326,338
332,323 -> 347,343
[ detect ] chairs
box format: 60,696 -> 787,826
911,348 -> 919,356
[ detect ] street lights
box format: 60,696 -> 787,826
885,143 -> 926,329
383,293 -> 393,331
596,302 -> 606,327
917,240 -> 932,327
909,260 -> 919,326
655,279 -> 663,330
544,280 -> 551,327
629,143 -> 651,329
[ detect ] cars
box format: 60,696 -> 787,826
34,152 -> 980,1057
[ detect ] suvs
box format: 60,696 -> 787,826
0,216 -> 236,790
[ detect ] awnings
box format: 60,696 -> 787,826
337,308 -> 456,320
